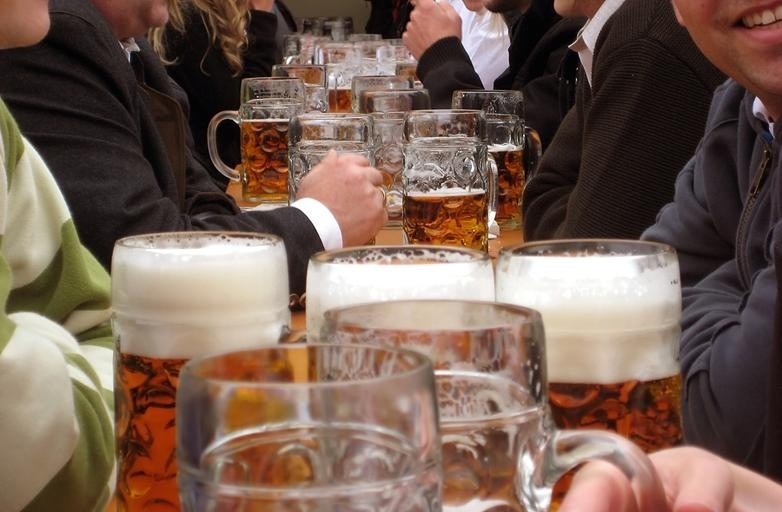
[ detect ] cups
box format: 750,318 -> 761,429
382,39 -> 404,46
272,64 -> 330,113
495,238 -> 684,512
452,89 -> 525,126
395,57 -> 420,81
402,108 -> 489,164
346,33 -> 382,43
375,45 -> 410,75
319,16 -> 354,41
207,98 -> 306,203
275,32 -> 301,63
287,139 -> 376,247
367,111 -> 404,230
302,40 -> 354,66
287,112 -> 375,148
325,62 -> 377,113
354,40 -> 385,59
313,298 -> 665,511
401,137 -> 499,255
110,231 -> 291,511
241,76 -> 306,114
174,342 -> 445,512
296,16 -> 329,37
359,89 -> 431,115
305,245 -> 494,425
286,35 -> 333,65
485,112 -> 542,232
350,76 -> 410,114
361,55 -> 376,63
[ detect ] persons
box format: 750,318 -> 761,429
521,1 -> 728,241
1,1 -> 388,310
638,1 -> 780,482
405,1 -> 562,113
556,446 -> 781,512
150,1 -> 279,167
447,0 -> 510,91
0,1 -> 117,512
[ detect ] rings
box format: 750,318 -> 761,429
377,185 -> 387,200
